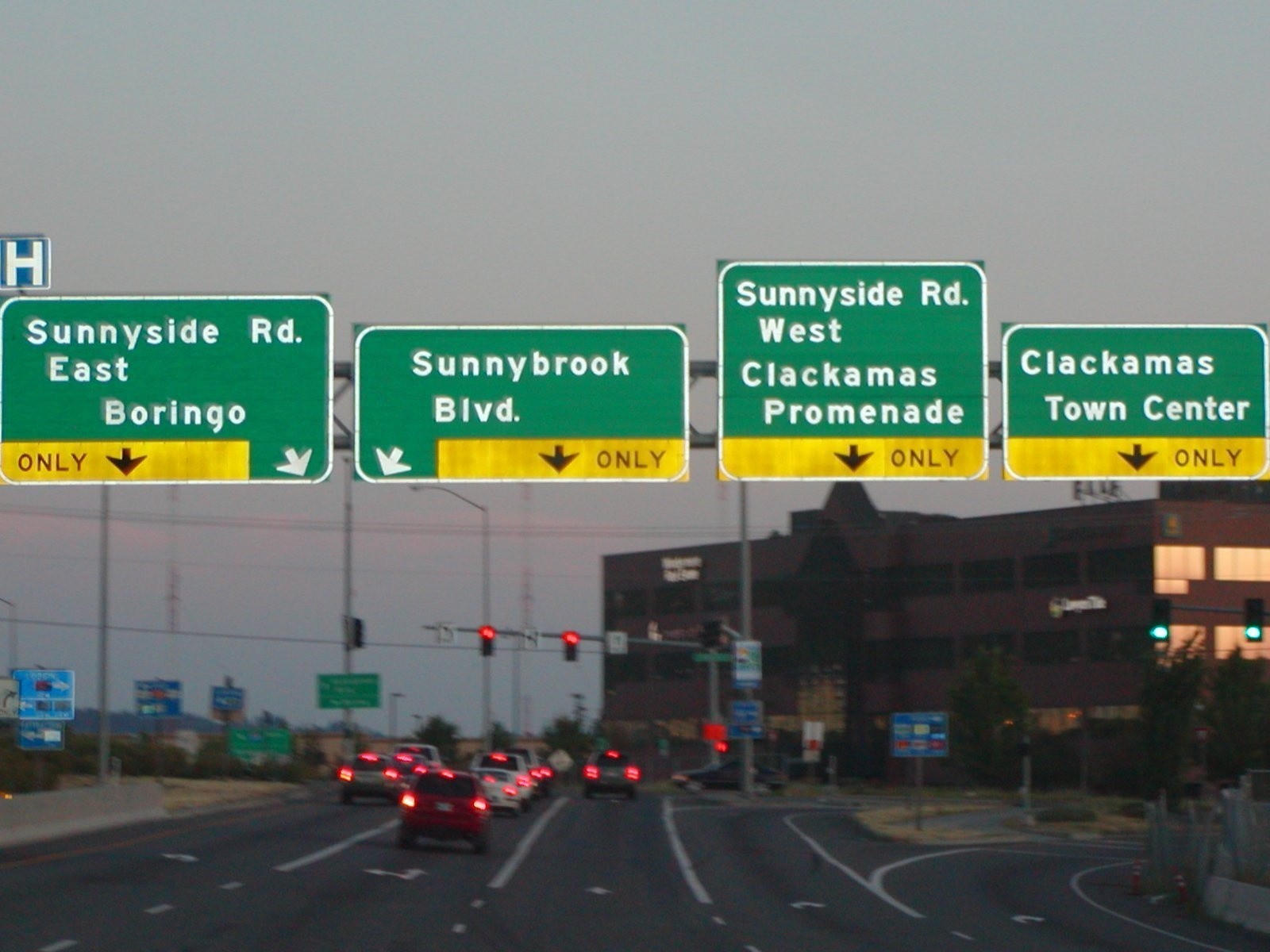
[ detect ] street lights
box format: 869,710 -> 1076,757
389,693 -> 408,748
407,484 -> 491,751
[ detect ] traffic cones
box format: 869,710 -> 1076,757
1170,866 -> 1191,907
1121,859 -> 1145,896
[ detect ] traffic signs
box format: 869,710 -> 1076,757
315,670 -> 384,709
1000,319 -> 1270,480
10,667 -> 76,722
134,681 -> 181,718
211,688 -> 247,710
716,256 -> 993,482
891,712 -> 952,758
225,728 -> 290,758
16,719 -> 66,751
727,700 -> 763,740
350,320 -> 691,488
0,292 -> 337,487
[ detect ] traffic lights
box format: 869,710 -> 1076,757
1246,598 -> 1265,643
563,627 -> 578,662
1150,598 -> 1175,643
479,624 -> 493,657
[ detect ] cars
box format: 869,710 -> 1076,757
674,758 -> 792,793
584,748 -> 640,801
337,742 -> 551,815
396,769 -> 496,855
